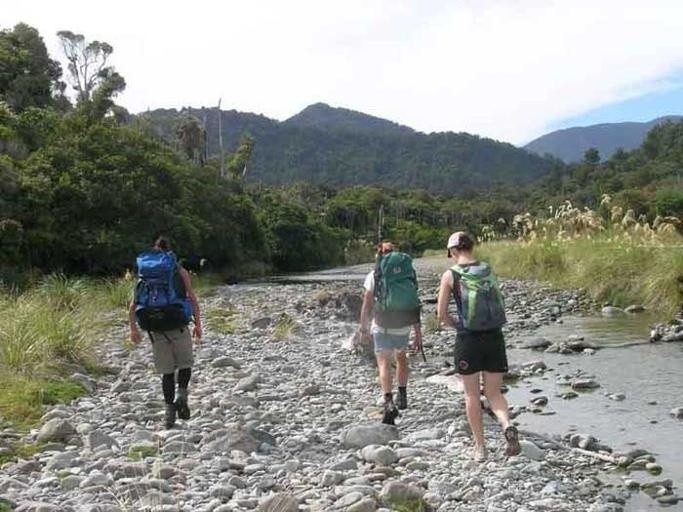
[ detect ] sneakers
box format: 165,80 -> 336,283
395,395 -> 407,408
504,426 -> 521,455
176,397 -> 189,418
382,407 -> 398,424
473,445 -> 488,461
165,403 -> 176,427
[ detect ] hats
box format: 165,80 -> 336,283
447,231 -> 473,257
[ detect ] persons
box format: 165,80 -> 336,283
437,231 -> 520,463
128,237 -> 203,429
356,242 -> 422,423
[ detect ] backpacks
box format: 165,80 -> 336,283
374,251 -> 419,328
133,249 -> 192,332
451,263 -> 506,330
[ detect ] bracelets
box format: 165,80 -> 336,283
129,321 -> 136,325
359,327 -> 368,334
